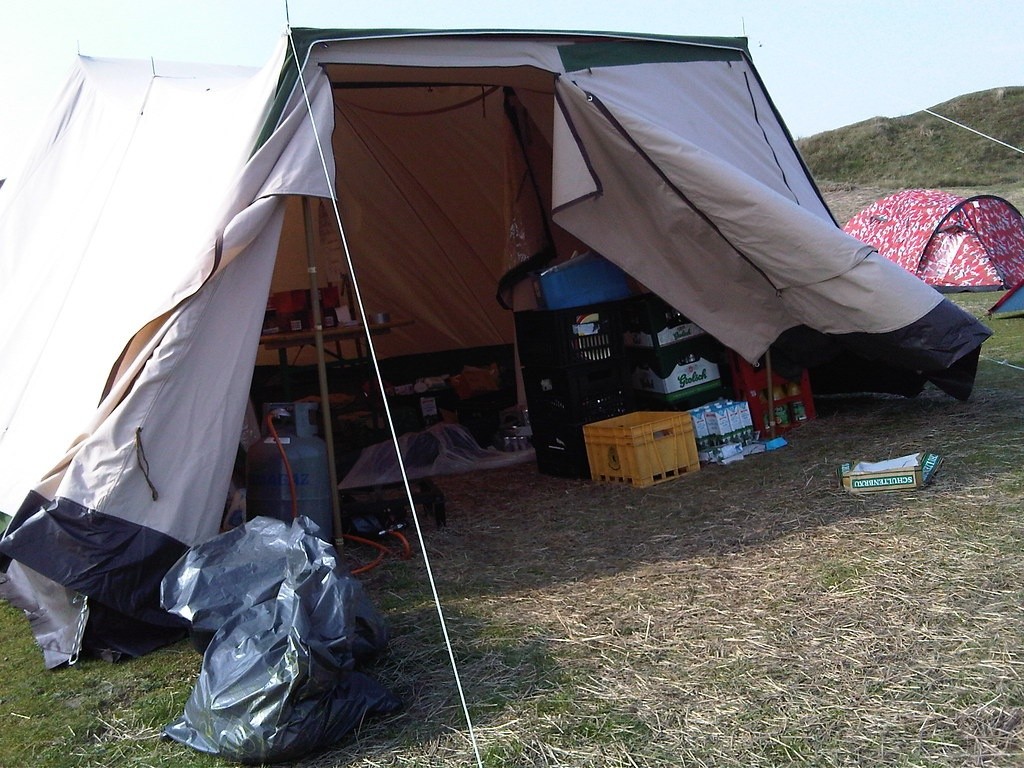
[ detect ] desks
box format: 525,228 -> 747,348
260,316 -> 416,430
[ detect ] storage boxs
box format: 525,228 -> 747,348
622,347 -> 728,411
511,301 -> 631,479
730,349 -> 817,440
583,411 -> 701,489
625,288 -> 708,350
530,249 -> 632,311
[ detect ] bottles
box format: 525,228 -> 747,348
756,381 -> 808,431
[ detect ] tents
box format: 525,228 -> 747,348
839,190 -> 1024,295
0,28 -> 992,672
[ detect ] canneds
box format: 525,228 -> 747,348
510,437 -> 519,450
503,437 -> 511,451
518,437 -> 526,449
